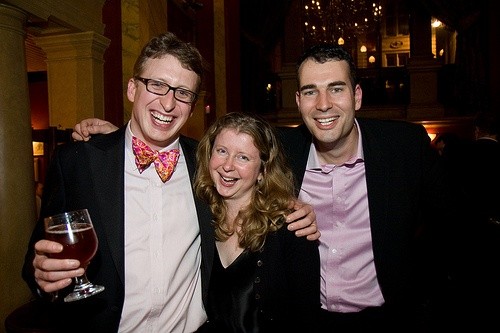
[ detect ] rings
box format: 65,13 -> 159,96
311,224 -> 317,228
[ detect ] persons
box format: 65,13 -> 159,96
272,42 -> 500,333
461,111 -> 500,162
21,33 -> 321,333
71,107 -> 323,333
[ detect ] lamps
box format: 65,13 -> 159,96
302,0 -> 382,63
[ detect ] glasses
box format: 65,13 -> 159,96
134,76 -> 199,104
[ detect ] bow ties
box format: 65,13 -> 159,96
132,136 -> 180,183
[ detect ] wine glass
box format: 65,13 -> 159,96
44,209 -> 105,302
